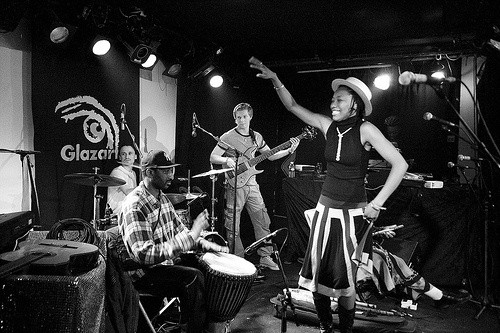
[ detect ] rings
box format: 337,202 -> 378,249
260,62 -> 262,65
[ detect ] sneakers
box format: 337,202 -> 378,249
259,256 -> 280,271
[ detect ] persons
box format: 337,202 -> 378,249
105,143 -> 137,210
209,102 -> 300,270
249,56 -> 409,333
384,116 -> 409,160
118,150 -> 228,333
370,224 -> 444,310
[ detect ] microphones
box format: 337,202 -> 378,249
446,161 -> 458,168
398,70 -> 457,84
191,116 -> 197,137
244,230 -> 278,256
423,111 -> 458,127
120,108 -> 125,131
457,153 -> 472,161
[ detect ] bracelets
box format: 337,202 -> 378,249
274,85 -> 284,89
370,201 -> 386,211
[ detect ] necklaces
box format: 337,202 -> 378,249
336,127 -> 352,161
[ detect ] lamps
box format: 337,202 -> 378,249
397,60 -> 415,85
38,12 -> 185,80
204,68 -> 224,88
372,65 -> 391,92
429,60 -> 446,80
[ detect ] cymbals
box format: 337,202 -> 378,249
165,195 -> 187,205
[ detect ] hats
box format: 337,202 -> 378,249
331,77 -> 372,116
141,149 -> 181,169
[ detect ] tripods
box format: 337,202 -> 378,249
455,124 -> 500,320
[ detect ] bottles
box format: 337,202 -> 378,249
288,161 -> 295,178
315,163 -> 322,174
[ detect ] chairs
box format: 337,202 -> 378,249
105,224 -> 183,333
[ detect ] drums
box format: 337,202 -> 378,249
198,250 -> 258,323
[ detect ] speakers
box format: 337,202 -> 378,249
378,238 -> 426,273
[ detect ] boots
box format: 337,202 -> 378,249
313,297 -> 334,333
338,297 -> 356,333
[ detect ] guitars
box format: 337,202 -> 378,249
222,125 -> 317,188
0,239 -> 99,287
